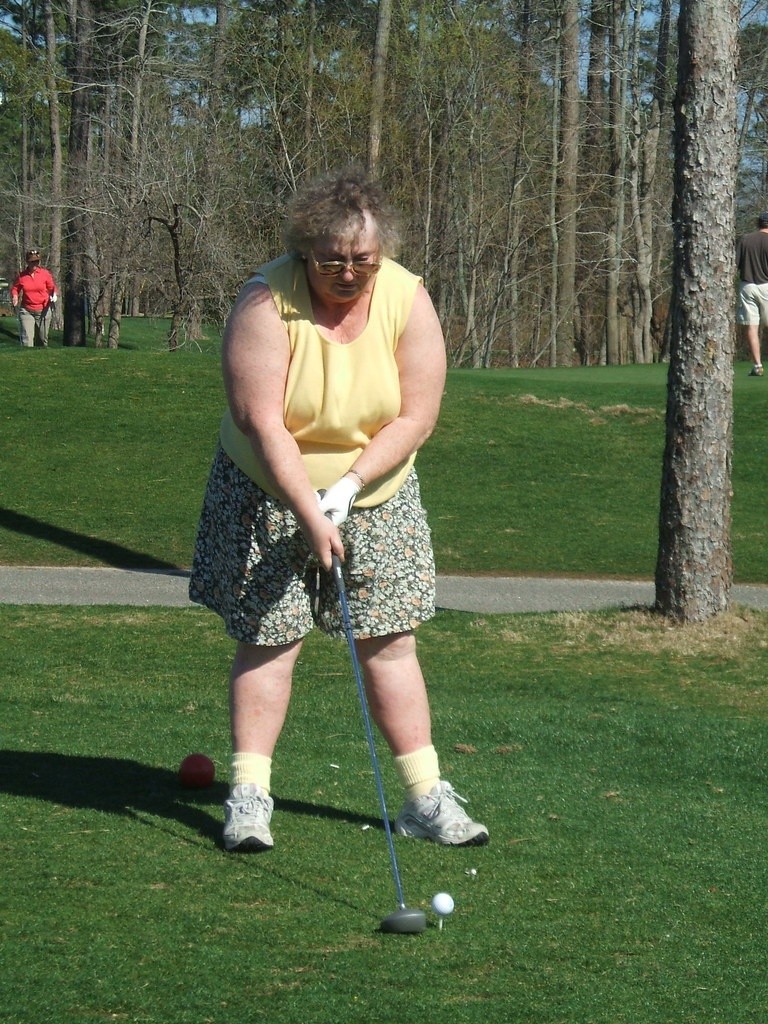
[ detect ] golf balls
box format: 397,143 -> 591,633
431,893 -> 454,916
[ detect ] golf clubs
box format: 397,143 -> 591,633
316,486 -> 426,933
11,305 -> 23,346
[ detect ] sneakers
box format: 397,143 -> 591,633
223,783 -> 274,853
394,781 -> 489,847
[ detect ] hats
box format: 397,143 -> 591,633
26,250 -> 41,261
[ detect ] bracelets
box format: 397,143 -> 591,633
342,471 -> 367,494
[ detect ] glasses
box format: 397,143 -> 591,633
309,247 -> 382,278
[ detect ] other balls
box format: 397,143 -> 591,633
178,754 -> 215,787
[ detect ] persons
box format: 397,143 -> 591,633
11,249 -> 58,347
188,168 -> 490,852
735,211 -> 768,377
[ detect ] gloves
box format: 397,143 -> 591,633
319,477 -> 362,527
50,292 -> 58,302
